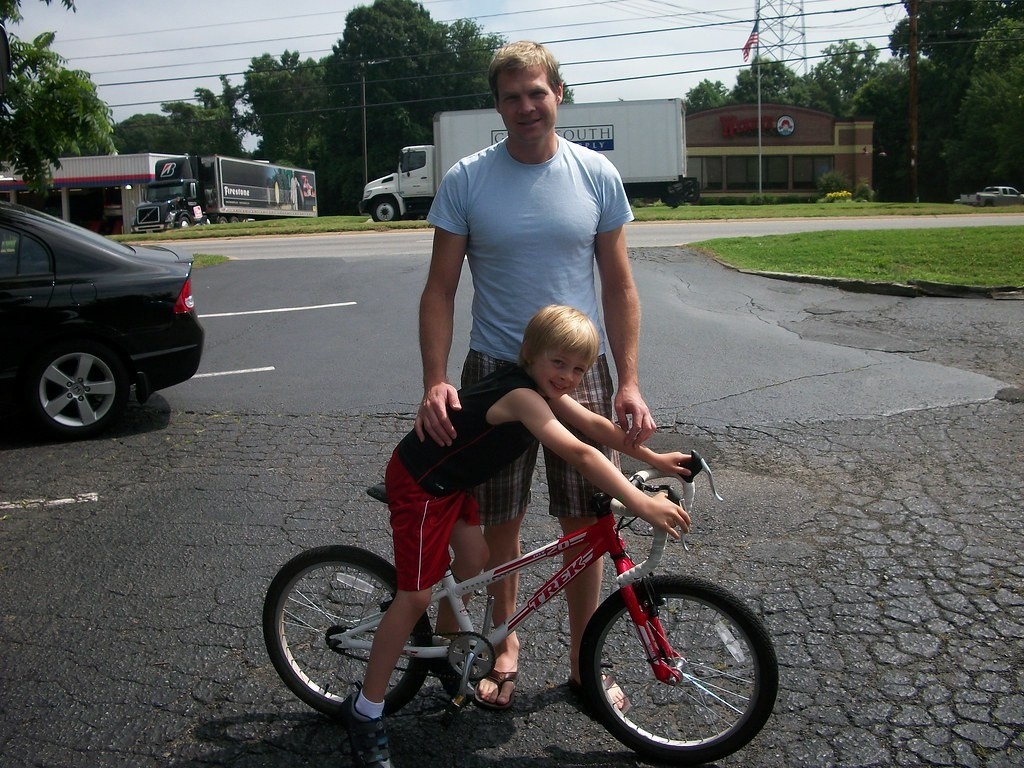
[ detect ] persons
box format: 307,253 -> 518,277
290,170 -> 300,211
335,303 -> 691,768
419,39 -> 658,714
302,176 -> 312,212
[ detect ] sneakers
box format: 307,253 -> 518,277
338,692 -> 394,768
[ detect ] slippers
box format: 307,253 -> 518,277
473,668 -> 519,711
567,672 -> 630,715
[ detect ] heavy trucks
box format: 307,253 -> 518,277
129,151 -> 318,234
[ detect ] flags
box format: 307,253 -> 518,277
742,22 -> 758,61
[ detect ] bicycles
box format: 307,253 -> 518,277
260,444 -> 779,764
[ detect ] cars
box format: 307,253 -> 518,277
975,186 -> 1024,198
0,199 -> 209,435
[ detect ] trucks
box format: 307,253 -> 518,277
361,96 -> 701,222
